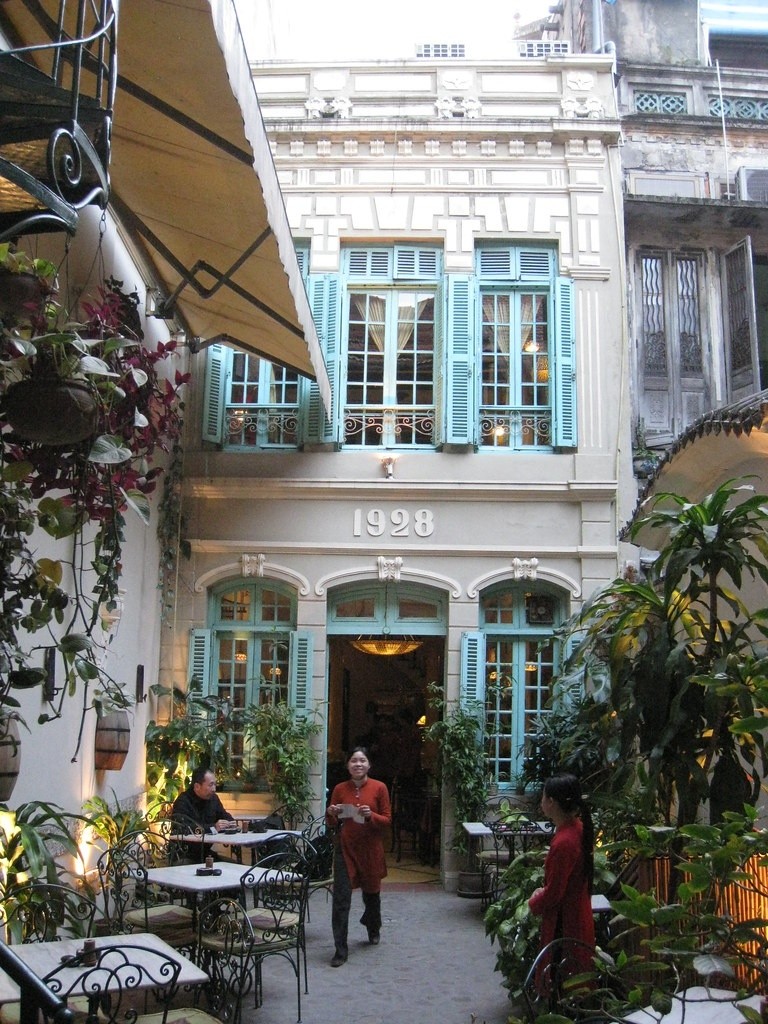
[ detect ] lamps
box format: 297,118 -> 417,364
86,869 -> 101,895
351,634 -> 424,655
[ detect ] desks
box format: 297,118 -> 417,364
462,820 -> 557,914
0,933 -> 209,1024
124,861 -> 303,997
169,828 -> 308,910
608,986 -> 768,1024
590,893 -> 611,913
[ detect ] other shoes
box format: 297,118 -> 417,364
210,907 -> 230,914
368,923 -> 380,945
331,955 -> 347,966
198,904 -> 208,912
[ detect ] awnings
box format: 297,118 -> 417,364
0,1 -> 327,387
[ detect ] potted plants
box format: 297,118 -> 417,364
484,771 -> 508,795
0,207 -> 189,763
511,769 -> 533,796
240,756 -> 259,792
417,675 -> 508,897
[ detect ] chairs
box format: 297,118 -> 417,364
477,796 -> 608,1024
0,801 -> 335,1024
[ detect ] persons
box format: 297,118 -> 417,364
527,773 -> 596,993
324,746 -> 392,969
168,766 -> 241,864
